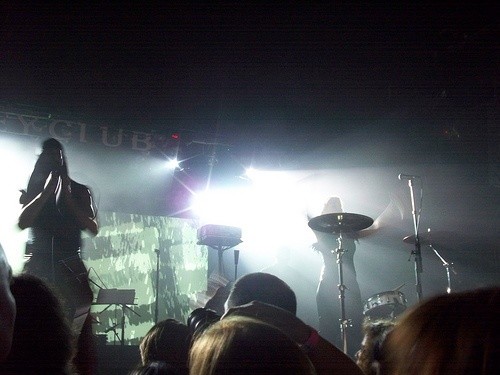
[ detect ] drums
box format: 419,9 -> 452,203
359,287 -> 407,318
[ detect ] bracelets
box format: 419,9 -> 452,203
299,326 -> 319,354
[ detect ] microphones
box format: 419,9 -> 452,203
398,174 -> 416,180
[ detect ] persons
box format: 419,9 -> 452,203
17,136 -> 99,374
303,194 -> 406,364
0,247 -> 500,375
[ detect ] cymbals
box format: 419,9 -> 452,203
307,211 -> 375,236
401,230 -> 460,244
